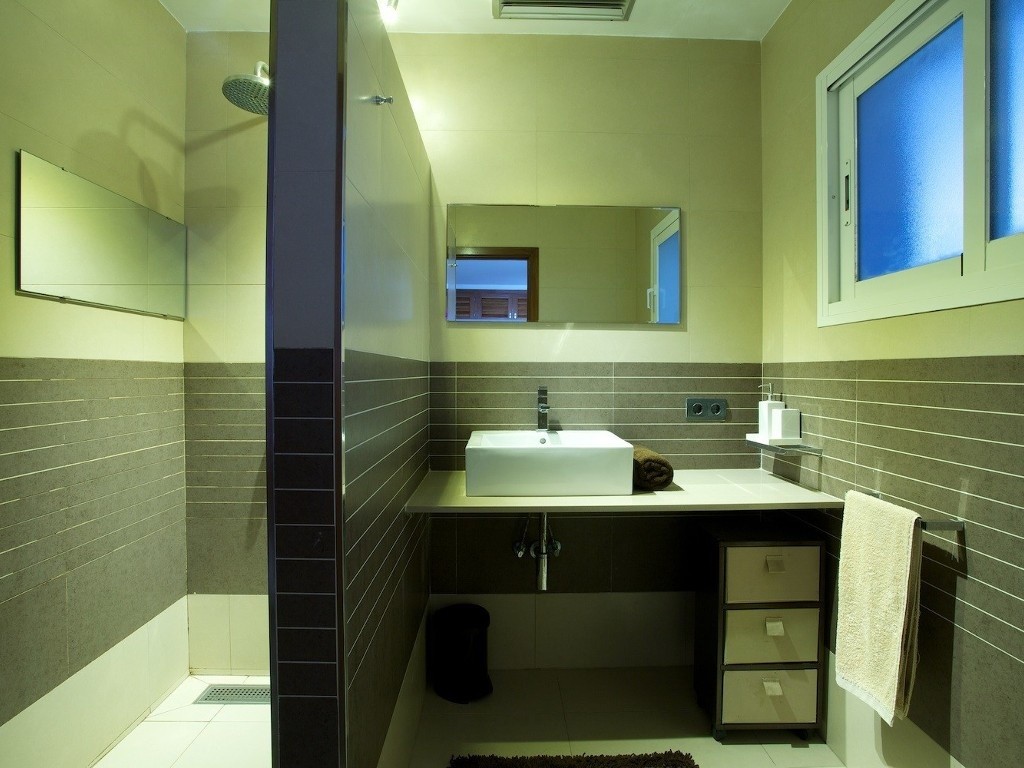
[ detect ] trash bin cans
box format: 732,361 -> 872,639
425,603 -> 494,704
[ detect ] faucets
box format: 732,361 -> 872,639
221,72 -> 269,115
535,385 -> 550,432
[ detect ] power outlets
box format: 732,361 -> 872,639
685,398 -> 727,422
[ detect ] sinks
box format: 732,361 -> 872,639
465,430 -> 634,497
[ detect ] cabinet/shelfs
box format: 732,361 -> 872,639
715,513 -> 830,734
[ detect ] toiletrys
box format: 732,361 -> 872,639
772,409 -> 800,439
758,383 -> 785,436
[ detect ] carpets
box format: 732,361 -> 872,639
446,748 -> 697,768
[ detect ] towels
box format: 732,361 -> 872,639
835,489 -> 923,728
631,442 -> 674,491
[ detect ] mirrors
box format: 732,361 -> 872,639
16,148 -> 187,324
446,202 -> 681,325
